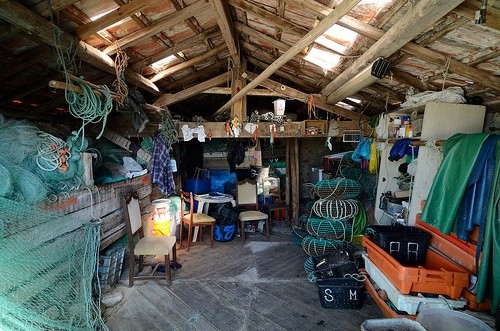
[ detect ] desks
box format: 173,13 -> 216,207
192,192 -> 240,243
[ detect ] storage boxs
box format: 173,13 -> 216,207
94,247 -> 125,286
312,200 -> 490,320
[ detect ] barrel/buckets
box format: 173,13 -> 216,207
152,199 -> 171,236
416,309 -> 494,331
186,178 -> 210,213
360,318 -> 428,331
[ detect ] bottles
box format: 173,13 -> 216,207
388,122 -> 393,138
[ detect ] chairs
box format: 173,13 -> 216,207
262,177 -> 290,228
180,189 -> 216,252
122,191 -> 176,288
236,178 -> 270,245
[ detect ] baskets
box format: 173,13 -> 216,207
311,248 -> 358,279
366,222 -> 431,265
315,274 -> 367,309
